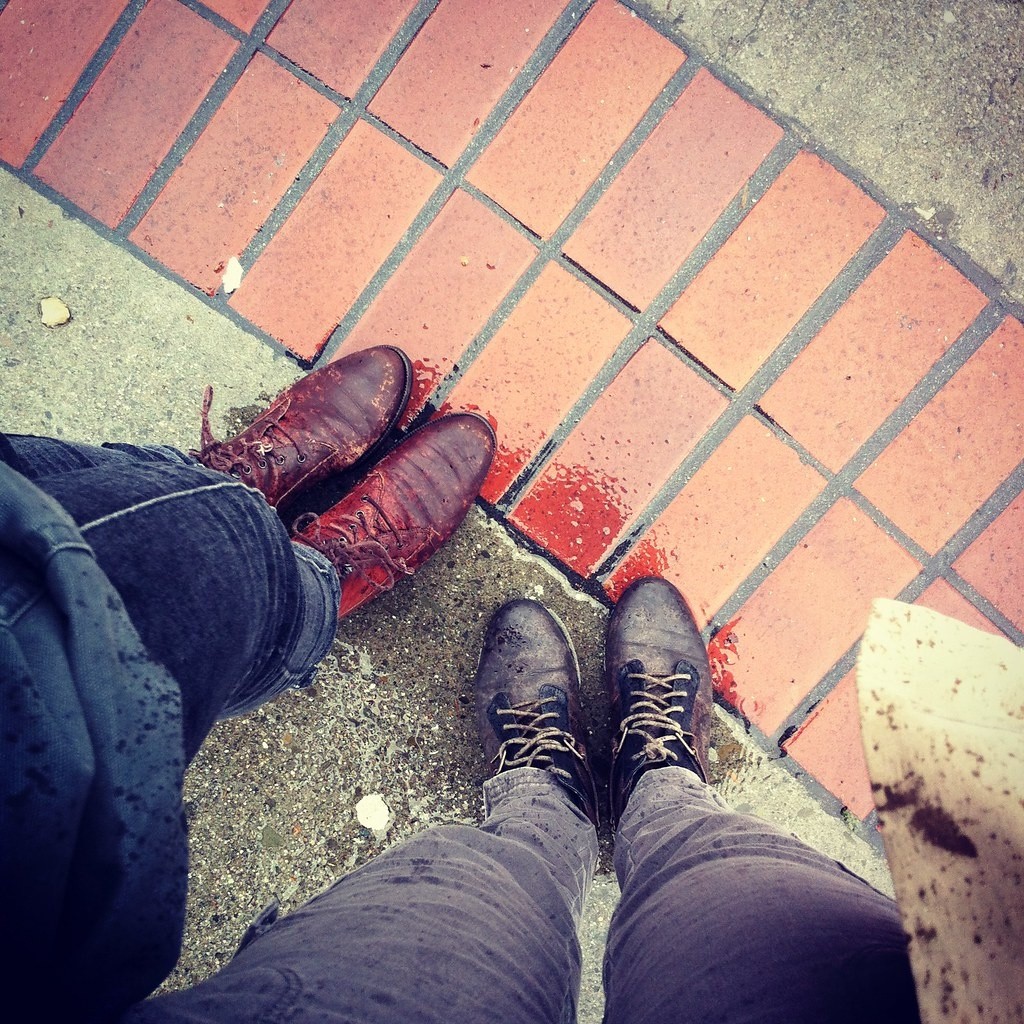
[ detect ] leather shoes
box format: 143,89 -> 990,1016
609,575 -> 704,782
195,339 -> 499,618
472,600 -> 591,794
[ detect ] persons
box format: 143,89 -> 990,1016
0,344 -> 497,1024
109,576 -> 921,1024
